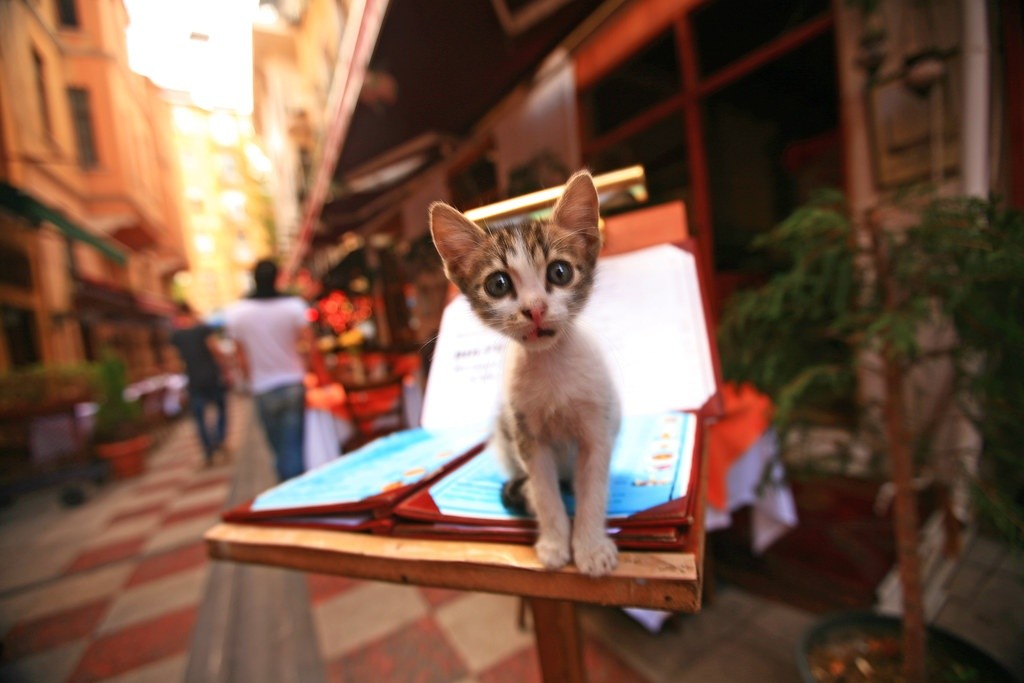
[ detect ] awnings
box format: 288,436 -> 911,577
0,180 -> 126,264
276,0 -> 614,307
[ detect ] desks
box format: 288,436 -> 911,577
205,412 -> 708,683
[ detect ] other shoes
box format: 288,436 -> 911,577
201,443 -> 221,466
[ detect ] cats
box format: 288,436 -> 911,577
427,170 -> 619,578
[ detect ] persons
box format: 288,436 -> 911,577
223,259 -> 330,484
168,300 -> 231,470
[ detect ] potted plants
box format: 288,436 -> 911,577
92,359 -> 156,480
716,180 -> 1024,683
